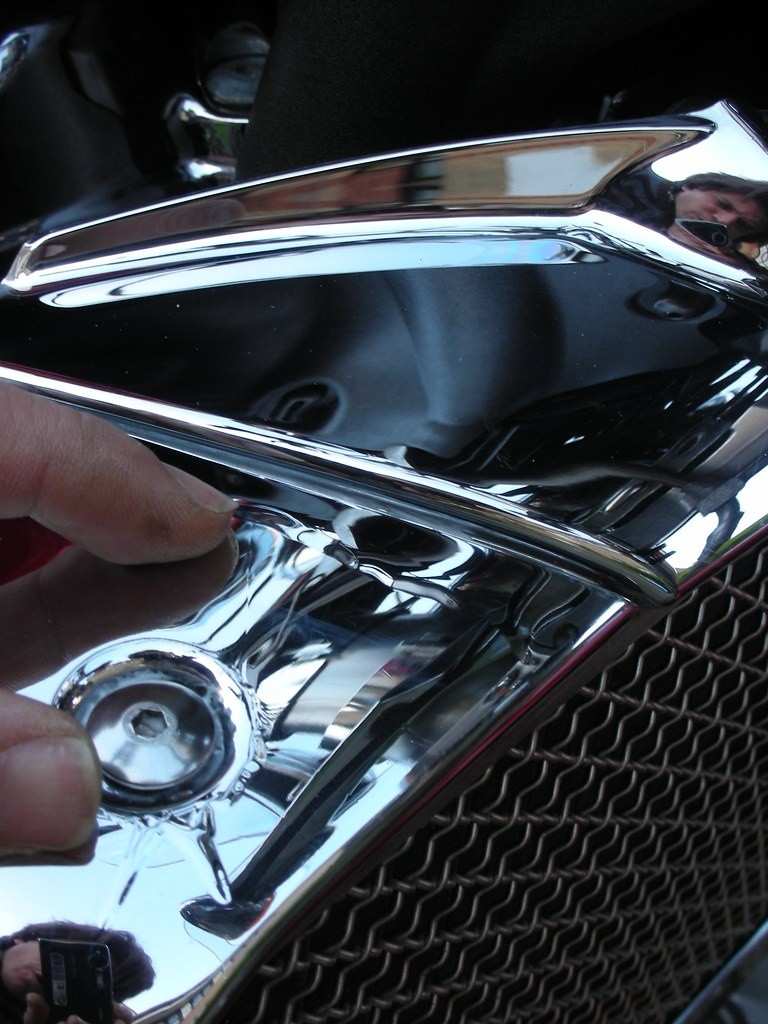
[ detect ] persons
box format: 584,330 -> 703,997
0,381 -> 239,851
0,922 -> 156,1024
596,161 -> 768,270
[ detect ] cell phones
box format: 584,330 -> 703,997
38,938 -> 113,1024
676,218 -> 730,251
